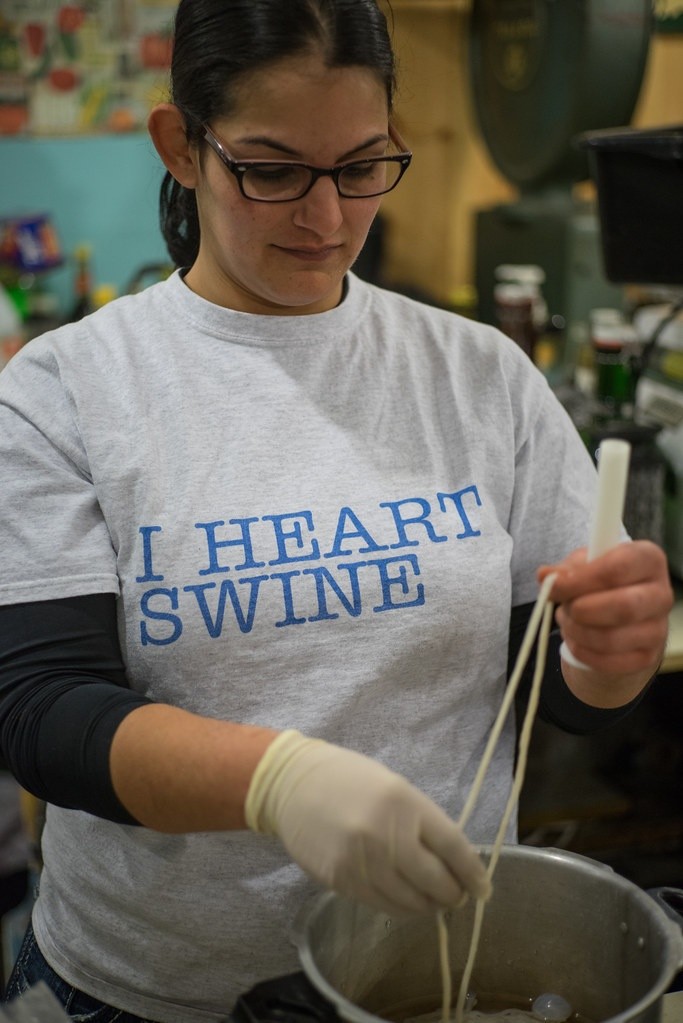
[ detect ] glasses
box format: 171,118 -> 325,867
173,99 -> 413,203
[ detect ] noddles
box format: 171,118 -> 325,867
378,574 -> 590,1023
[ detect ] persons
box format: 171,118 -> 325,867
0,0 -> 674,1023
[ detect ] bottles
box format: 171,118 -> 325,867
494,262 -> 546,368
64,237 -> 119,325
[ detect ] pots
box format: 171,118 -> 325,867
288,840 -> 683,1023
